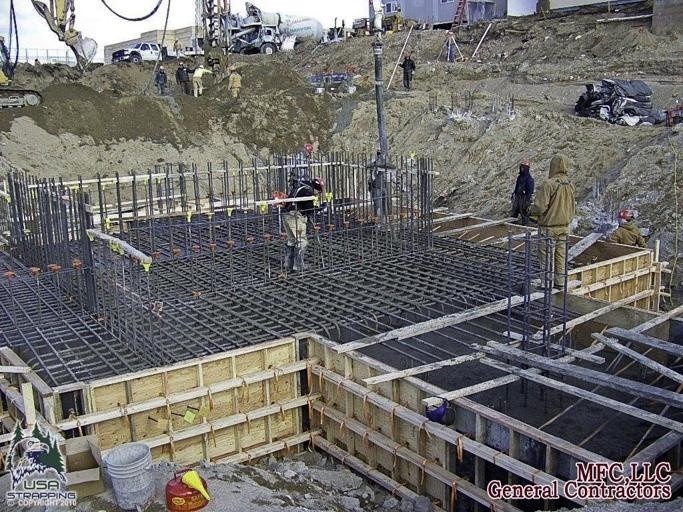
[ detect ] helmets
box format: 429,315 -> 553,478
616,208 -> 634,219
314,176 -> 325,188
521,160 -> 530,168
427,401 -> 455,426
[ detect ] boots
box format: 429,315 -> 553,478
283,243 -> 311,271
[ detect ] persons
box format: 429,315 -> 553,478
176,62 -> 196,96
154,66 -> 168,96
398,53 -> 415,91
228,65 -> 242,98
366,149 -> 396,225
34,58 -> 41,77
279,177 -> 325,271
173,38 -> 183,57
192,65 -> 212,98
511,160 -> 534,221
526,154 -> 575,290
604,207 -> 647,248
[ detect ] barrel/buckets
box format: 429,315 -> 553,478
105,442 -> 155,511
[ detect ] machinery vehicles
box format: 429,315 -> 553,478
0,1 -> 98,109
229,0 -> 324,55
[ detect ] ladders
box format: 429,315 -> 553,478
451,0 -> 466,35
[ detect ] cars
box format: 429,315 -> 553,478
111,40 -> 199,66
